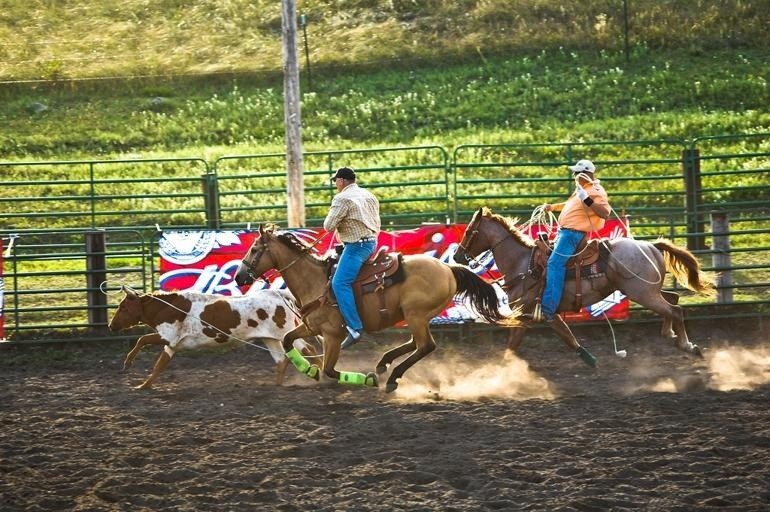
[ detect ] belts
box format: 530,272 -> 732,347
359,238 -> 376,242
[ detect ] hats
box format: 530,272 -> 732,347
568,159 -> 597,174
330,167 -> 355,182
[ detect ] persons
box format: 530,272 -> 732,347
517,158 -> 611,325
323,166 -> 382,348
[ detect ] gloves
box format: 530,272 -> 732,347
576,183 -> 590,200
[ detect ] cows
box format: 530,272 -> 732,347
106,283 -> 329,391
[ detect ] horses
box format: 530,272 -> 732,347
233,222 -> 523,394
452,205 -> 725,371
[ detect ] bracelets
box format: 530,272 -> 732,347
584,197 -> 595,208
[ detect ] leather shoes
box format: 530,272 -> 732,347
341,328 -> 367,349
522,313 -> 554,323
